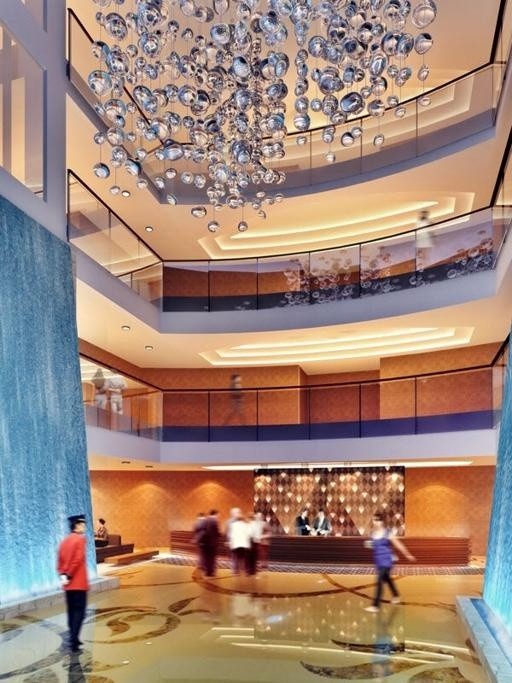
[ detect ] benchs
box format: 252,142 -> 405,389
104,549 -> 159,566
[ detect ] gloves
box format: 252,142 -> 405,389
59,575 -> 69,588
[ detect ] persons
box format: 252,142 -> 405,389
245,512 -> 267,576
294,508 -> 312,536
57,514 -> 89,652
222,372 -> 251,434
363,513 -> 416,613
94,519 -> 109,547
312,508 -> 333,536
224,507 -> 251,575
201,509 -> 223,577
193,512 -> 205,568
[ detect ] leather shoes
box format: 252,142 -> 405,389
69,646 -> 85,652
68,640 -> 84,646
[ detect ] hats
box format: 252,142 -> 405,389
67,514 -> 87,523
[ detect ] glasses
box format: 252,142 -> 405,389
371,518 -> 382,521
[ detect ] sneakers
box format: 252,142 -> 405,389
363,605 -> 381,613
391,596 -> 402,605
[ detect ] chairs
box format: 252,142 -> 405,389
94,534 -> 135,563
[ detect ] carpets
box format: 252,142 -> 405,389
154,555 -> 485,576
